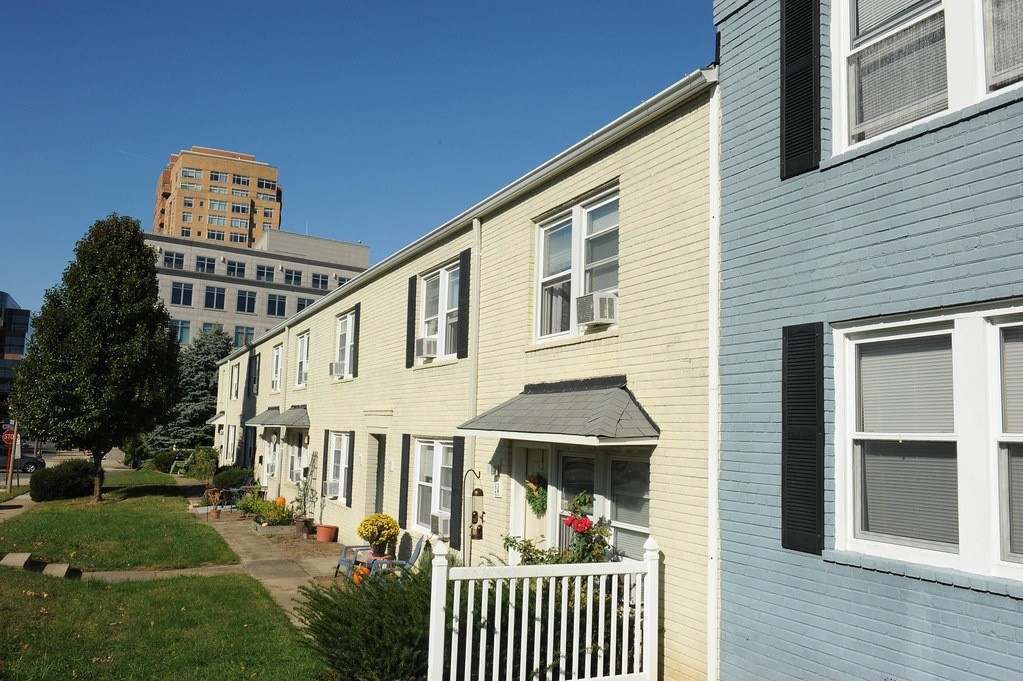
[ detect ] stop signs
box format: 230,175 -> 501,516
2,427 -> 17,444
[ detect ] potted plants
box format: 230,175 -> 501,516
291,452 -> 317,540
526,471 -> 547,518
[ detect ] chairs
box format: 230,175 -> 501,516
334,535 -> 425,582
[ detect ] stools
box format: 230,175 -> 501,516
366,554 -> 393,578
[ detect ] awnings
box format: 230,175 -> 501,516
244,406 -> 280,427
264,405 -> 310,428
459,384 -> 660,446
205,411 -> 226,425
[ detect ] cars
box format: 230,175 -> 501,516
0,446 -> 46,473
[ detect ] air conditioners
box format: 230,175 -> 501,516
267,463 -> 275,473
299,371 -> 307,383
252,384 -> 257,393
576,294 -> 618,326
290,470 -> 301,482
271,380 -> 278,390
322,480 -> 340,497
414,337 -> 438,357
429,510 -> 450,539
329,362 -> 346,376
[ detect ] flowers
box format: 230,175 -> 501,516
357,513 -> 400,544
210,492 -> 223,510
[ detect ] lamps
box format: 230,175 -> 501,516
461,468 -> 483,566
220,428 -> 224,435
304,435 -> 309,444
271,434 -> 277,444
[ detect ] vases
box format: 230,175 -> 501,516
211,508 -> 220,518
316,525 -> 339,542
369,543 -> 387,557
